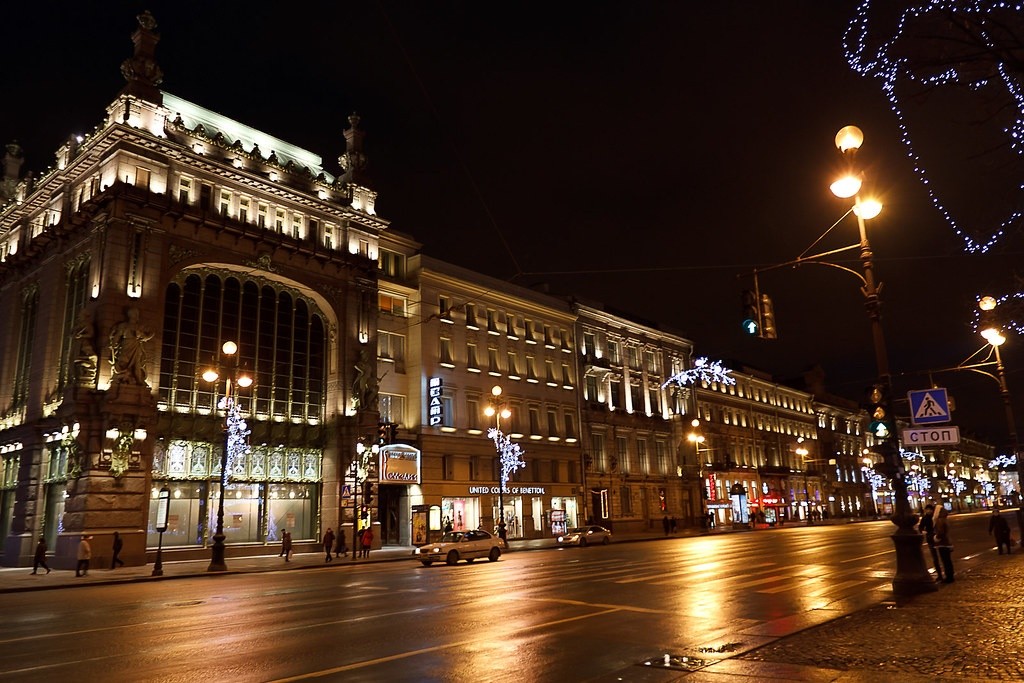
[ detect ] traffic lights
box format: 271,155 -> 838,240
867,382 -> 895,441
738,283 -> 765,340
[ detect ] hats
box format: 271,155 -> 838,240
992,506 -> 1000,513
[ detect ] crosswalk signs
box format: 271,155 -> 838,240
907,387 -> 952,426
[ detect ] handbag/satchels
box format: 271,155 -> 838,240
288,550 -> 292,559
1010,539 -> 1017,546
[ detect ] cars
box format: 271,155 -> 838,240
556,524 -> 612,547
414,529 -> 506,567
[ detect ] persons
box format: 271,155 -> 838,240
794,509 -> 829,521
113,307 -> 155,386
110,532 -> 124,569
751,510 -> 765,523
919,504 -> 955,583
704,512 -> 715,532
445,521 -> 452,536
278,529 -> 293,562
358,526 -> 374,558
354,360 -> 380,411
663,515 -> 676,535
31,539 -> 51,575
334,529 -> 349,558
989,509 -> 1011,554
323,527 -> 336,562
76,535 -> 91,577
587,515 -> 593,526
69,309 -> 94,382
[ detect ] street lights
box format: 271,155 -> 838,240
686,418 -> 715,530
977,293 -> 1024,500
825,122 -> 944,597
794,436 -> 812,526
483,384 -> 513,546
200,338 -> 256,572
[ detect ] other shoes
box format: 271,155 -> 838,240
31,571 -> 36,575
120,563 -> 124,567
935,576 -> 955,585
76,573 -> 88,577
47,569 -> 50,574
278,555 -> 282,557
109,567 -> 115,570
325,558 -> 333,563
345,555 -> 348,557
285,559 -> 290,562
336,556 -> 340,558
